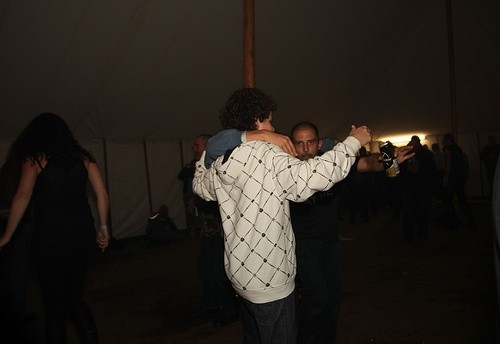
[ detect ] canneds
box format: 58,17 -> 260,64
379,141 -> 401,178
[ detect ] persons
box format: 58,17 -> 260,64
187,134 -> 242,327
191,87 -> 371,344
242,120 -> 417,344
336,134 -> 500,247
0,112 -> 111,344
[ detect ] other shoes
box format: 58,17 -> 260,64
84,330 -> 98,344
19,305 -> 37,323
343,214 -> 477,247
191,307 -> 223,318
213,314 -> 238,325
